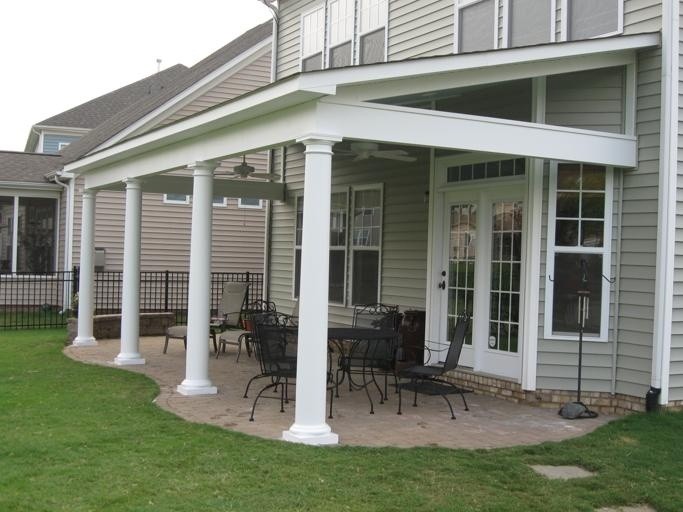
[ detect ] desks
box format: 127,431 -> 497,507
288,327 -> 400,414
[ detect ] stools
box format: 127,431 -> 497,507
164,326 -> 218,355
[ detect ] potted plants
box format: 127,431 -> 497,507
243,310 -> 256,330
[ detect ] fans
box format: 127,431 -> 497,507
213,154 -> 282,184
330,140 -> 417,164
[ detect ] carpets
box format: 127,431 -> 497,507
388,381 -> 473,396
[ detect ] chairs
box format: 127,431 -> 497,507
215,301 -> 262,359
338,303 -> 398,400
247,299 -> 296,392
394,311 -> 472,419
209,282 -> 252,352
247,313 -> 336,421
